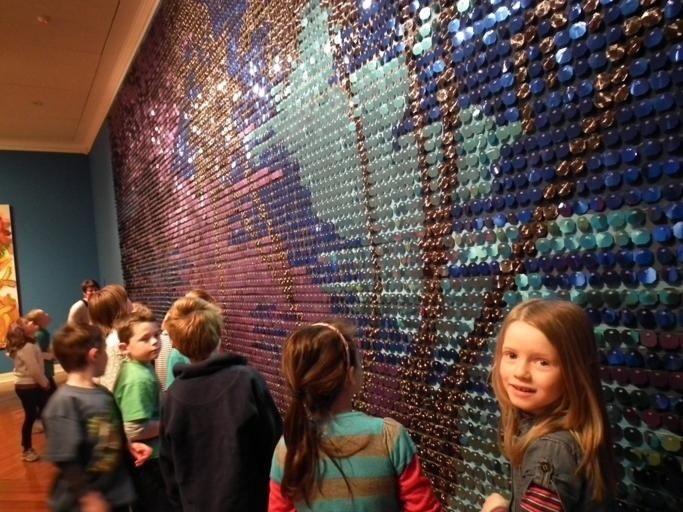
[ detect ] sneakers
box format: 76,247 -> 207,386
20,449 -> 39,462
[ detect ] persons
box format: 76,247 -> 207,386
5,279 -> 193,462
480,298 -> 613,512
39,323 -> 152,512
165,290 -> 222,423
262,321 -> 447,511
155,291 -> 283,512
112,306 -> 164,510
